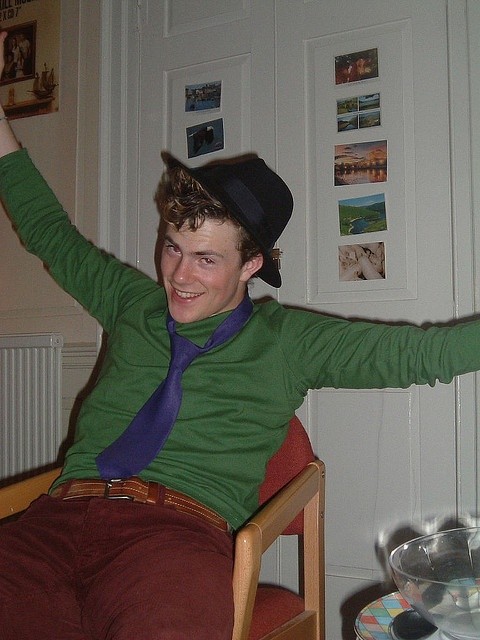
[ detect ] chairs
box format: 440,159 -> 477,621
1,414 -> 325,640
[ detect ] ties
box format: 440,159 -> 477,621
95,286 -> 253,481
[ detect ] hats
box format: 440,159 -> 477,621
161,151 -> 293,288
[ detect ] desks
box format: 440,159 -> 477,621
351,572 -> 480,640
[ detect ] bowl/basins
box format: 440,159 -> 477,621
390,527 -> 480,639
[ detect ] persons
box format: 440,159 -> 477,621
0,105 -> 480,639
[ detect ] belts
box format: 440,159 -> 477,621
49,477 -> 228,533
